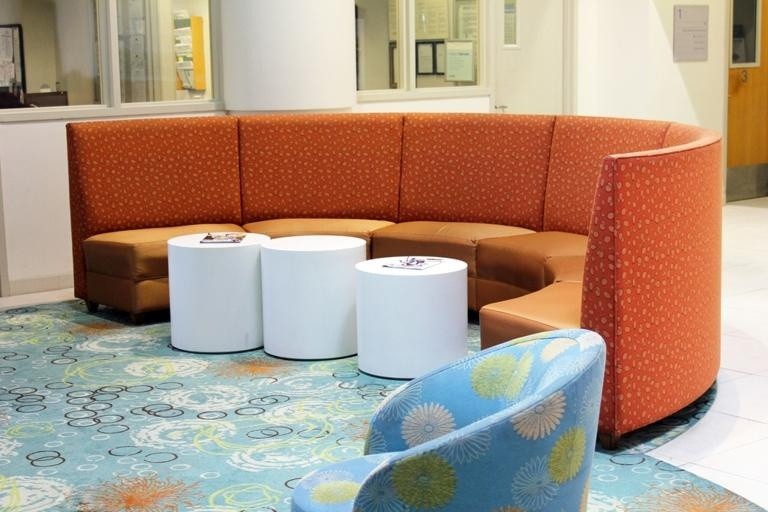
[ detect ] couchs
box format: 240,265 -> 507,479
65,113 -> 722,452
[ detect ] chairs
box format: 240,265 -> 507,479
291,327 -> 605,512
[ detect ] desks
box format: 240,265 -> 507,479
261,235 -> 368,362
357,256 -> 467,380
168,232 -> 270,355
